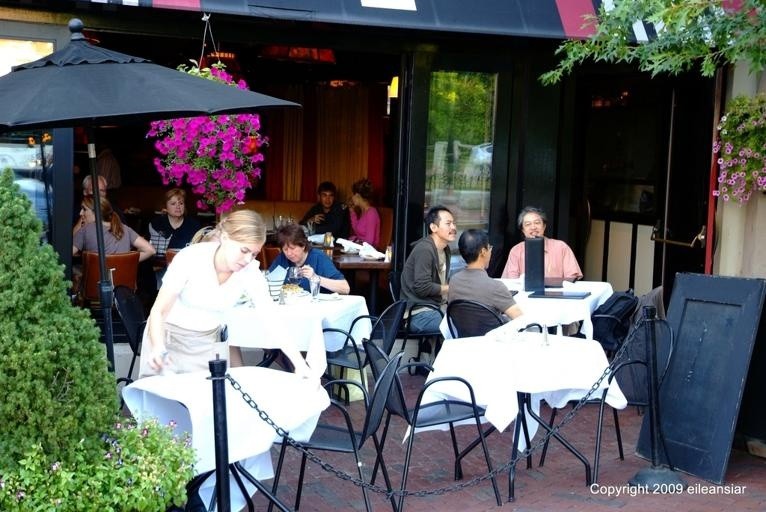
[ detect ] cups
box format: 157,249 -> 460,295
341,198 -> 354,210
324,232 -> 333,247
289,267 -> 303,279
309,280 -> 320,297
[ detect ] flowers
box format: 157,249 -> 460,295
141,58 -> 270,215
703,90 -> 766,207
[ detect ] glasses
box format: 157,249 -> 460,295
480,245 -> 493,252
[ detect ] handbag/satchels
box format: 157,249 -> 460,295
333,350 -> 369,401
591,288 -> 639,357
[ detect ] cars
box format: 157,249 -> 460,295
0,147 -> 58,233
468,136 -> 494,179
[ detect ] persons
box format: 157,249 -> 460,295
341,177 -> 381,247
138,210 -> 323,385
447,228 -> 522,334
299,182 -> 348,237
70,173 -> 108,235
144,188 -> 216,290
71,194 -> 156,262
267,222 -> 351,292
501,206 -> 583,280
399,206 -> 458,335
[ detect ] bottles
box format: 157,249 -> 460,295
279,288 -> 294,305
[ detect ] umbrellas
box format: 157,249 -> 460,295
0,17 -> 303,393
